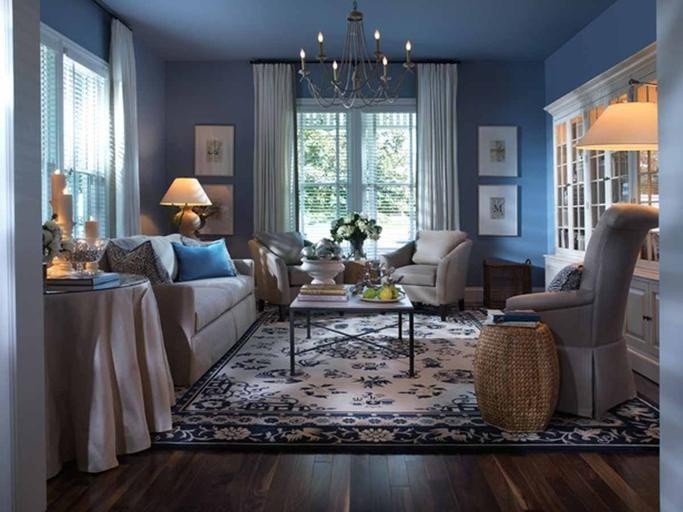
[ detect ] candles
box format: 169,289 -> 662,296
56,193 -> 73,223
85,220 -> 99,242
51,174 -> 65,215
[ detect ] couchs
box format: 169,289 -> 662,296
505,203 -> 660,417
248,238 -> 345,321
379,230 -> 473,321
99,233 -> 257,386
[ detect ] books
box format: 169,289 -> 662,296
482,305 -> 542,330
44,271 -> 121,292
299,282 -> 350,302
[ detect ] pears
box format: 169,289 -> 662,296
379,286 -> 393,300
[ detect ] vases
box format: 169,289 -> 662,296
43,264 -> 48,294
349,239 -> 365,255
300,256 -> 346,285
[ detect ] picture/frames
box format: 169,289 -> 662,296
477,125 -> 519,177
476,184 -> 519,237
193,123 -> 236,178
197,183 -> 234,236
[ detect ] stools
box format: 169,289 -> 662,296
473,324 -> 560,434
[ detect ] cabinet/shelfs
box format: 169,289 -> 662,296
543,253 -> 570,292
482,258 -> 532,307
553,107 -> 587,256
621,269 -> 660,404
607,79 -> 660,274
582,99 -> 607,262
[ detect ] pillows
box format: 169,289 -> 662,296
142,233 -> 181,280
171,240 -> 237,282
181,236 -> 237,275
412,230 -> 467,265
107,239 -> 171,283
253,231 -> 304,264
546,262 -> 583,292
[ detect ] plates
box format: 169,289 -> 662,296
359,292 -> 405,302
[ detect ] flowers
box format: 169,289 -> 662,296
42,220 -> 63,266
330,211 -> 382,243
300,239 -> 342,260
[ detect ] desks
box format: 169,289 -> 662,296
43,273 -> 177,479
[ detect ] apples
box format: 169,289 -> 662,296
392,287 -> 399,299
363,287 -> 377,300
376,285 -> 385,298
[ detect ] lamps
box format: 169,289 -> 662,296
575,77 -> 657,152
298,0 -> 415,111
159,177 -> 212,239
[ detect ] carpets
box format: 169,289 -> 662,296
148,303 -> 660,454
